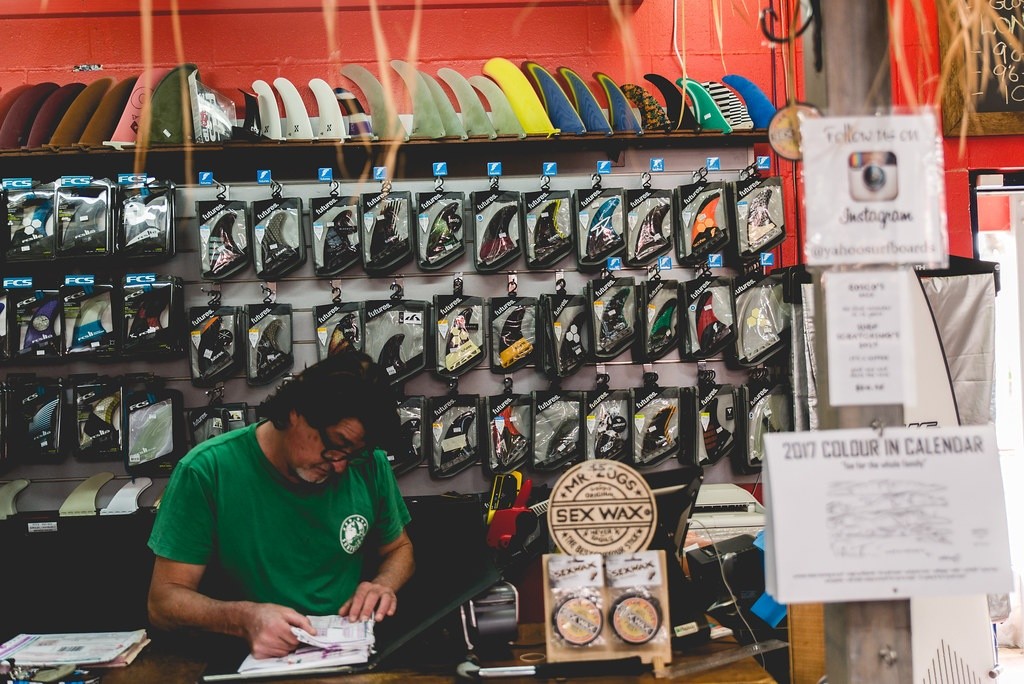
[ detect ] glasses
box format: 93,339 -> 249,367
320,429 -> 357,463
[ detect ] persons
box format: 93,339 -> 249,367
146,347 -> 417,660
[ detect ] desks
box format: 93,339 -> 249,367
95,622 -> 783,684
